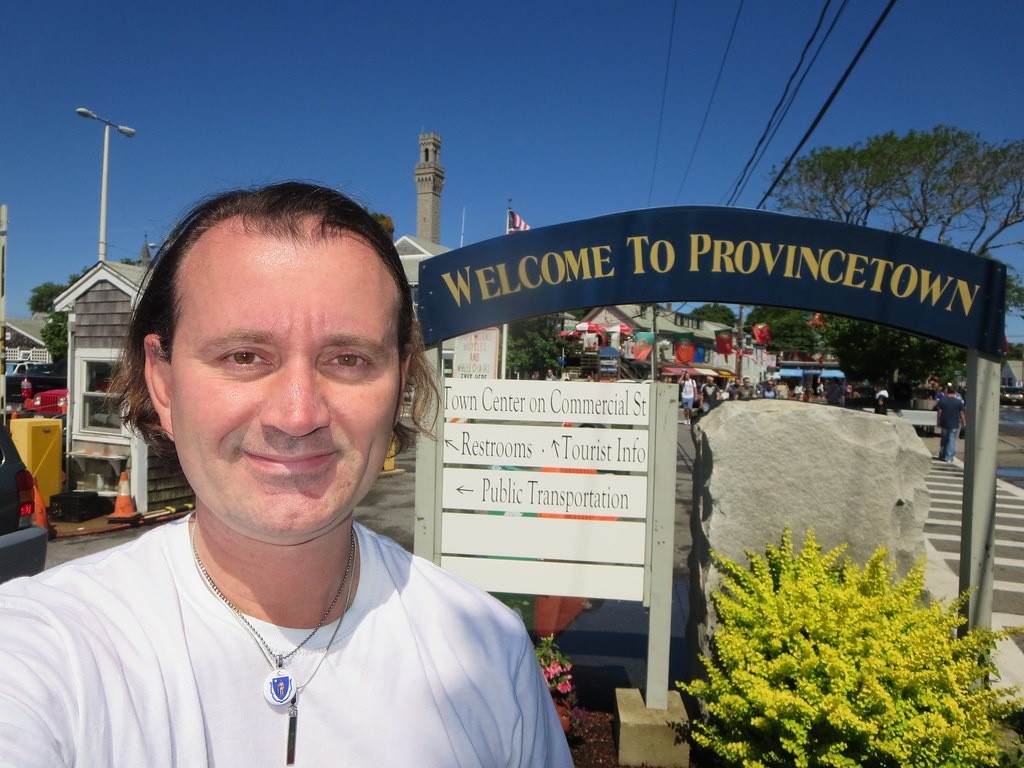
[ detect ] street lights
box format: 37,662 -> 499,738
76,108 -> 137,262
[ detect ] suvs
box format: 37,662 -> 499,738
16,372 -> 110,431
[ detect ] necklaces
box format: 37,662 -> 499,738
189,514 -> 357,765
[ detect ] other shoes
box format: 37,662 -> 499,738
940,456 -> 946,461
684,419 -> 691,425
947,458 -> 954,463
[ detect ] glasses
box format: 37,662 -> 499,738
709,380 -> 714,382
768,383 -> 773,386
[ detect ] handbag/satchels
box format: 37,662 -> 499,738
959,425 -> 966,439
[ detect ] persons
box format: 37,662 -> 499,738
524,366 -> 967,465
0,176 -> 574,768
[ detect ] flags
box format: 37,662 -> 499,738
508,210 -> 532,233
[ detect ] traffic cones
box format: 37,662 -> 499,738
105,472 -> 142,519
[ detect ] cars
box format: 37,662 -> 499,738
7,364 -> 67,403
0,425 -> 48,584
5,360 -> 44,376
1000,387 -> 1024,406
